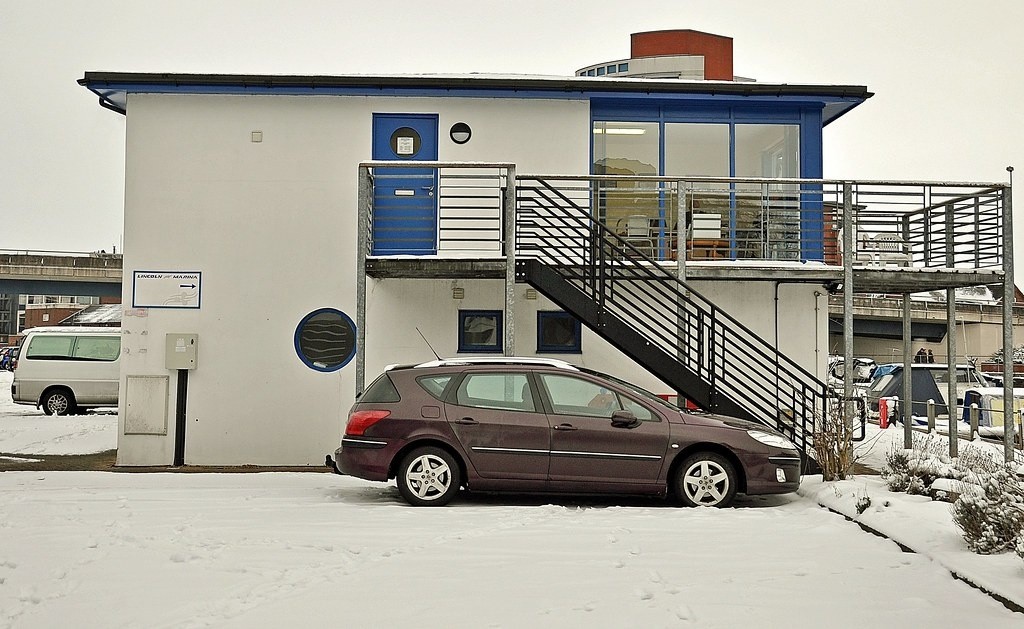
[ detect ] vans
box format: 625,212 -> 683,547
10,326 -> 121,417
885,363 -> 990,405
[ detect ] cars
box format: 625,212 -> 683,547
0,346 -> 20,371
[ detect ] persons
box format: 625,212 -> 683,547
928,349 -> 934,363
914,348 -> 927,363
673,199 -> 707,232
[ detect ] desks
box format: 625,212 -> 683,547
653,232 -> 726,261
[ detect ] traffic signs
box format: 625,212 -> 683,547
131,270 -> 201,310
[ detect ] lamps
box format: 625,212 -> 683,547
593,127 -> 647,135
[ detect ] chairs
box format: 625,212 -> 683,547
837,224 -> 917,267
622,214 -> 654,260
744,217 -> 787,260
521,384 -> 534,411
737,212 -> 771,258
686,214 -> 721,261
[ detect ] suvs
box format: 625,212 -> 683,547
324,355 -> 802,509
